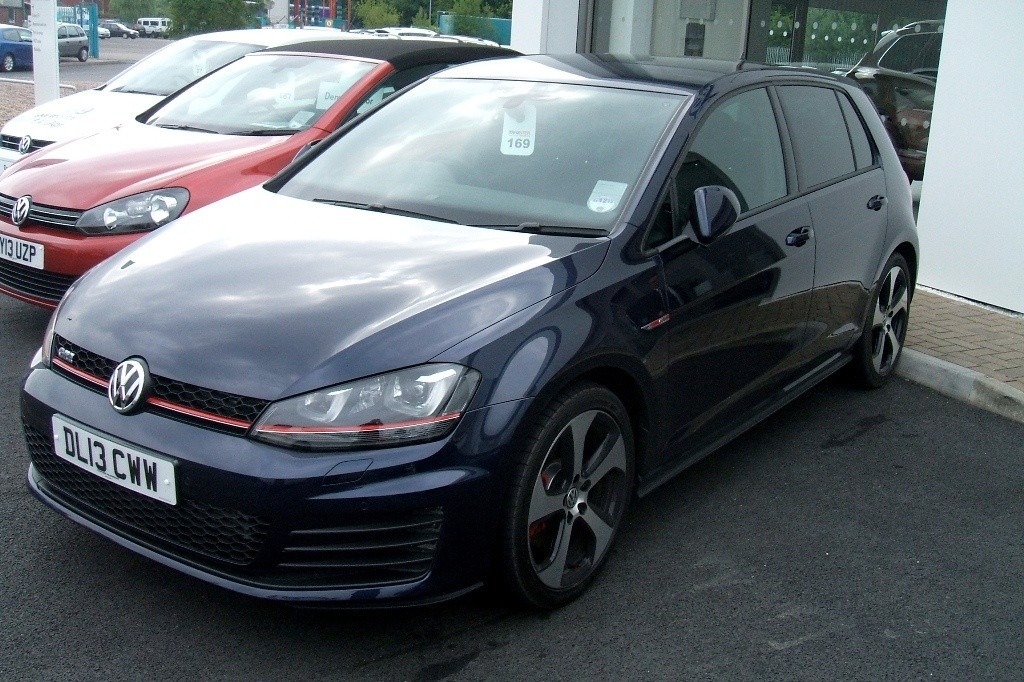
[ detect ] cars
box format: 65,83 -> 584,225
0,24 -> 34,72
0,27 -> 524,312
82,19 -> 139,39
55,22 -> 89,62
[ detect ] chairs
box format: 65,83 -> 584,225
675,112 -> 751,218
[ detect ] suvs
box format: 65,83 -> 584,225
845,20 -> 947,178
18,53 -> 922,618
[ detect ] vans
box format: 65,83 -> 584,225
136,18 -> 174,37
